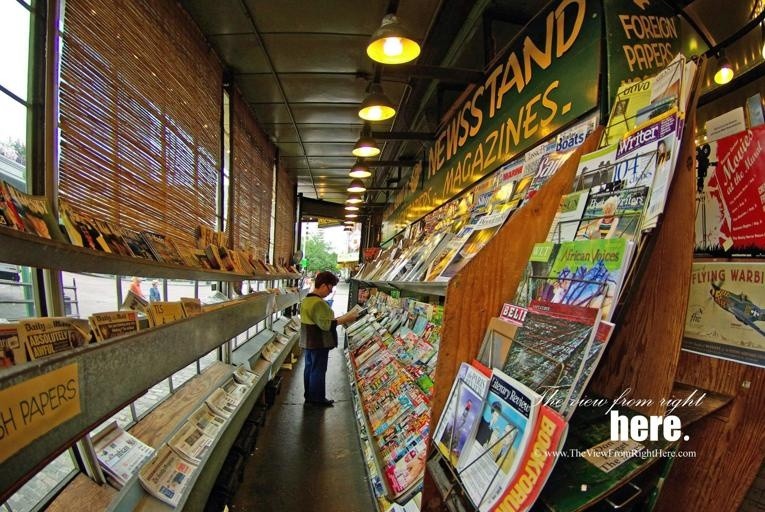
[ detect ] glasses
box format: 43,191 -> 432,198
327,284 -> 332,293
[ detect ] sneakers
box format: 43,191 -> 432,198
305,400 -> 334,407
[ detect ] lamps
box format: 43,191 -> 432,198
346,11 -> 423,205
712,47 -> 735,86
